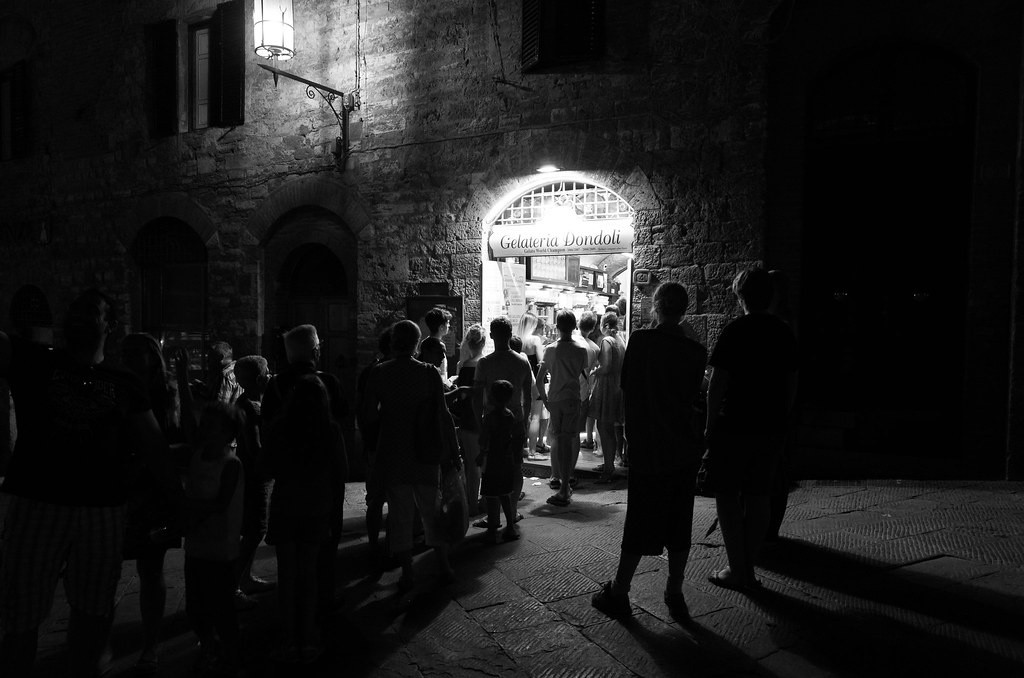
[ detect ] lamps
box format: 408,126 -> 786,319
248,0 -> 356,176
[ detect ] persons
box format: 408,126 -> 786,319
706,261 -> 802,590
592,282 -> 707,609
0,287 -> 624,677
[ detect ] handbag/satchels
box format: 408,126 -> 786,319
432,410 -> 469,545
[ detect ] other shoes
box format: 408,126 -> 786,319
473,519 -> 502,527
592,464 -> 604,471
663,575 -> 690,621
231,590 -> 260,608
397,576 -> 415,593
134,656 -> 159,674
546,496 -> 570,507
432,564 -> 466,592
594,468 -> 615,484
592,580 -> 633,618
244,576 -> 276,595
518,492 -> 525,500
267,642 -> 299,664
550,480 -> 561,488
300,645 -> 326,664
479,530 -> 497,544
500,527 -> 520,540
707,569 -> 758,592
568,477 -> 577,486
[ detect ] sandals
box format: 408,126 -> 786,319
580,439 -> 594,449
535,444 -> 550,453
